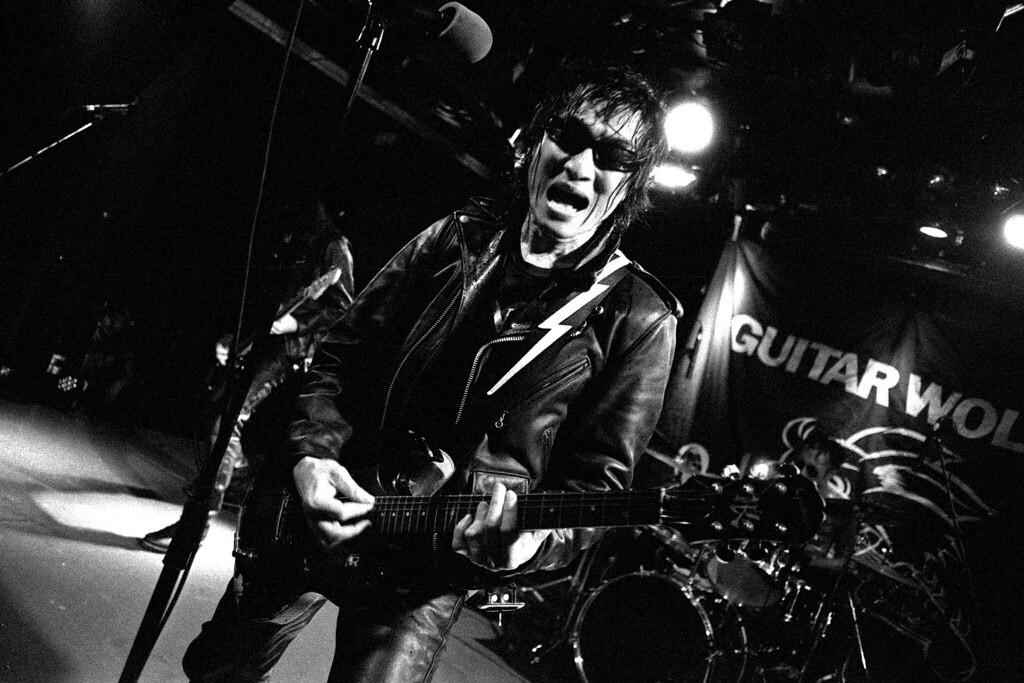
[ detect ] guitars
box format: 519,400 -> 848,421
232,424 -> 827,624
202,265 -> 343,400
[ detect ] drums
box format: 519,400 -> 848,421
571,567 -> 747,683
575,526 -> 698,597
705,542 -> 801,611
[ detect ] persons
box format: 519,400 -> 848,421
180,63 -> 685,683
142,195 -> 352,552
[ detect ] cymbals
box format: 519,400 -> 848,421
644,448 -> 708,490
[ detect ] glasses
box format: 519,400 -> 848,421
546,114 -> 642,173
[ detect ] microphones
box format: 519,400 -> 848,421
82,98 -> 148,115
912,416 -> 959,473
369,0 -> 495,68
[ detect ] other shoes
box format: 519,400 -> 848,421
139,521 -> 208,554
